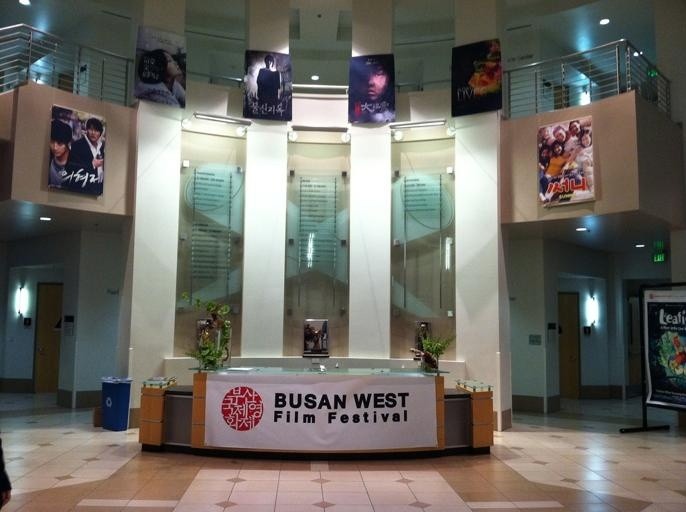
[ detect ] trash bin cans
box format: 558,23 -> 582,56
101,377 -> 133,431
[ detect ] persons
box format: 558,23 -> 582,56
0,438 -> 12,512
537,121 -> 594,204
50,116 -> 105,194
350,57 -> 394,123
256,55 -> 281,108
134,49 -> 185,108
